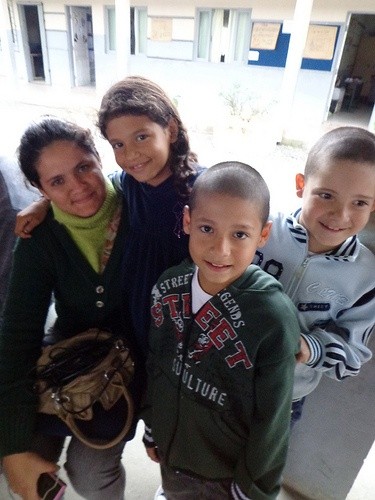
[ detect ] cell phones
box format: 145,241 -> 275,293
37,472 -> 66,500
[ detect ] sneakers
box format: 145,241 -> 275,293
154,484 -> 167,500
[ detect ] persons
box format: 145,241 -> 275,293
14,78 -> 209,383
247,127 -> 375,437
0,114 -> 139,500
136,161 -> 302,500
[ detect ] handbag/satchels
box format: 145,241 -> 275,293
35,324 -> 142,451
